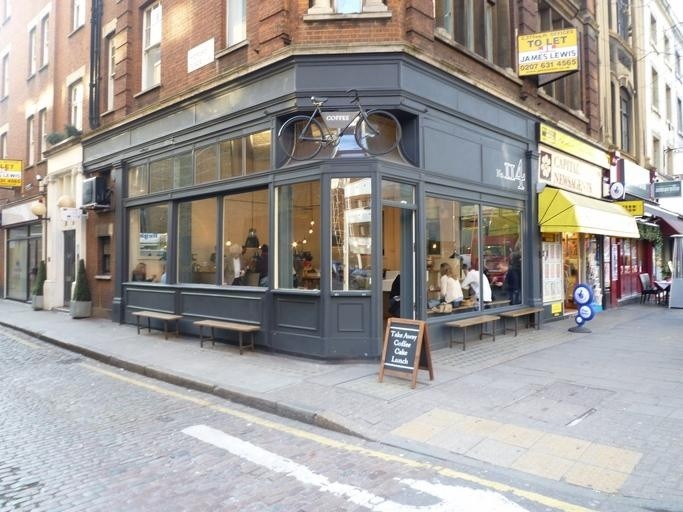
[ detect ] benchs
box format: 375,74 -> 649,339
193,320 -> 260,355
132,311 -> 184,340
500,307 -> 545,336
445,315 -> 501,351
427,299 -> 511,318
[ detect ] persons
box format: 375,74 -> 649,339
458,263 -> 492,304
132,262 -> 157,282
192,224 -> 318,291
389,272 -> 400,318
439,263 -> 464,308
503,250 -> 523,305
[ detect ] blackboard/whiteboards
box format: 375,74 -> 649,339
381,317 -> 425,380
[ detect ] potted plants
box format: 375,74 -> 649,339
31,260 -> 46,309
69,259 -> 93,318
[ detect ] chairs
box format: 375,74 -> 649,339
638,273 -> 664,304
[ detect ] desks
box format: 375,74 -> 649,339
654,281 -> 672,305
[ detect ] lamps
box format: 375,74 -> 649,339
244,192 -> 260,250
31,199 -> 50,221
56,195 -> 75,208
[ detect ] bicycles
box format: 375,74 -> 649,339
277,88 -> 403,161
